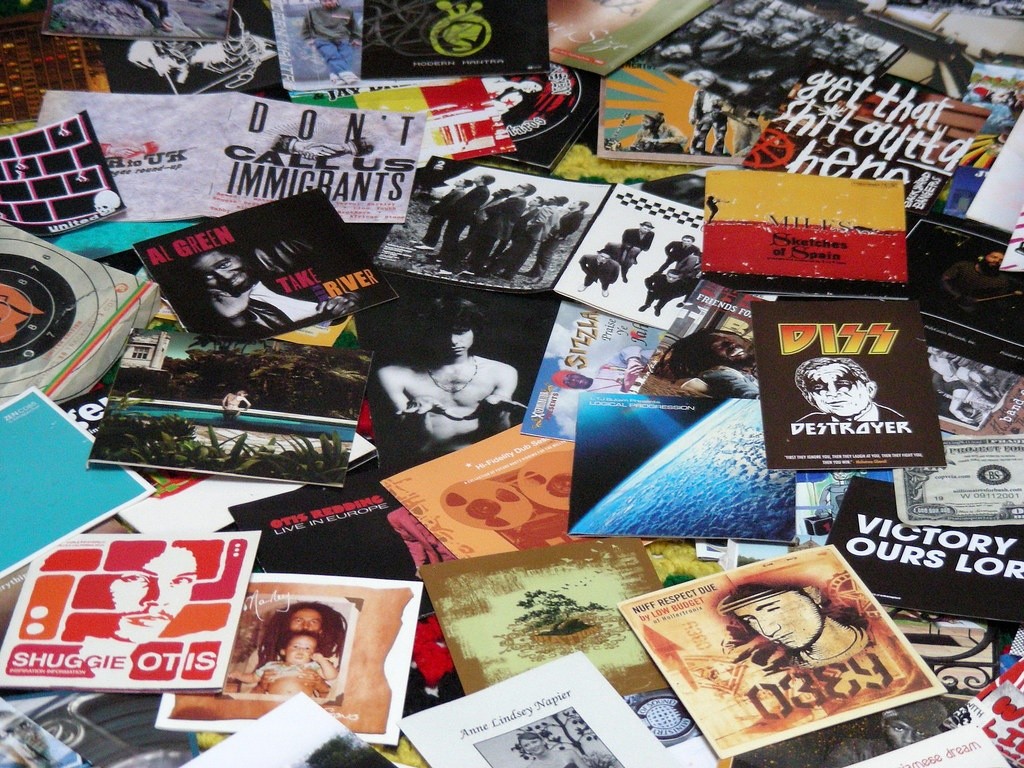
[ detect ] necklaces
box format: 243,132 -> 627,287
424,358 -> 477,393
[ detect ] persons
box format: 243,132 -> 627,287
520,732 -> 590,768
229,601 -> 348,696
638,235 -> 701,316
553,346 -> 655,394
828,699 -> 948,768
578,221 -> 655,296
193,243 -> 384,334
646,1 -> 885,117
669,330 -> 759,399
930,353 -> 1001,423
965,74 -> 1024,112
0,710 -> 61,768
629,91 -> 760,158
376,296 -> 518,464
551,619 -> 584,632
222,391 -> 251,422
129,0 -> 173,32
413,175 -> 589,282
302,0 -> 362,86
